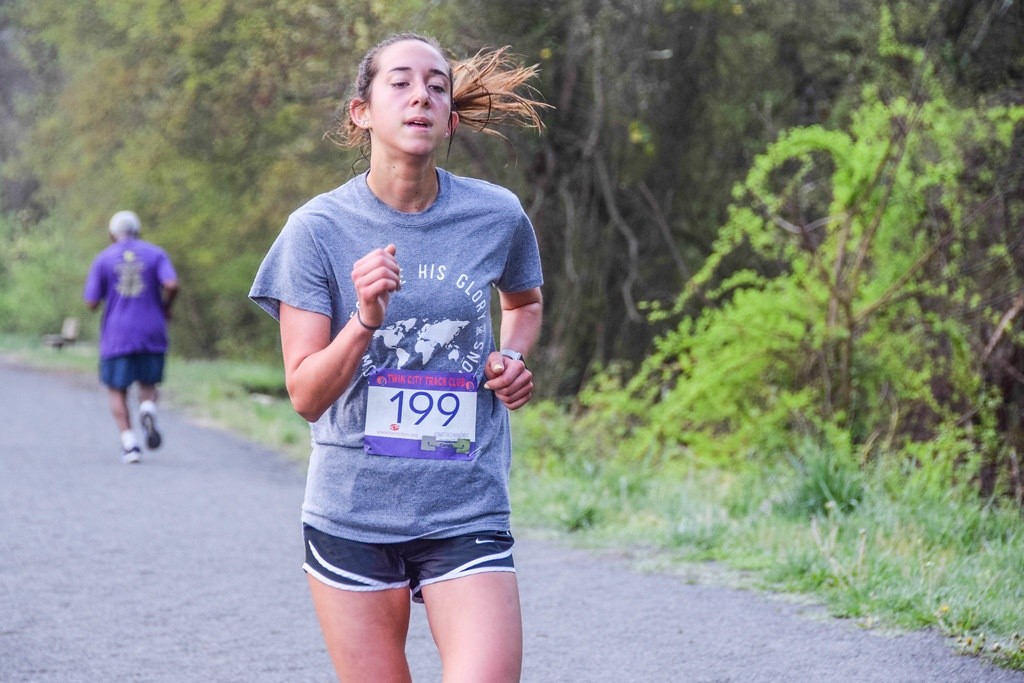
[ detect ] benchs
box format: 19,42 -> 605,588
45,317 -> 79,347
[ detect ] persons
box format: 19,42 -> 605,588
247,30 -> 544,682
83,210 -> 184,465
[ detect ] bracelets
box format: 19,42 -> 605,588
354,299 -> 384,331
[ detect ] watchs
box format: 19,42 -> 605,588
500,349 -> 528,370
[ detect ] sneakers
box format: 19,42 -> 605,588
121,410 -> 161,465
